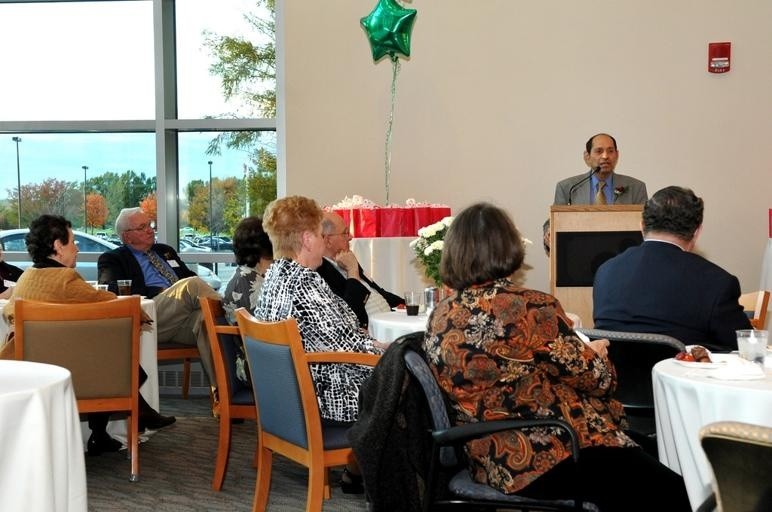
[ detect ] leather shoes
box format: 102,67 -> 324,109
213,401 -> 242,423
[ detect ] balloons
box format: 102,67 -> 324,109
360,0 -> 417,64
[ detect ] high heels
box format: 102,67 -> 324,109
88,432 -> 121,455
138,404 -> 176,434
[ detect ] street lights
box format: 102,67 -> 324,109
82,163 -> 89,234
12,136 -> 22,228
207,159 -> 213,234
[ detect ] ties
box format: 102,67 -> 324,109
144,249 -> 178,285
595,181 -> 608,206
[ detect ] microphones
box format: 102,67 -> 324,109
566,165 -> 602,203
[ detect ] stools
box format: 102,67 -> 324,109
155,341 -> 215,403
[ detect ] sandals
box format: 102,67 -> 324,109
339,467 -> 365,494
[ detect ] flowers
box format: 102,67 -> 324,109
408,216 -> 531,291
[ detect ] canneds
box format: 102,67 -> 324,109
424,286 -> 439,312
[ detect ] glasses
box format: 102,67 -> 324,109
125,221 -> 150,232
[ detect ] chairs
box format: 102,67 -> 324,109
199,298 -> 261,490
403,351 -> 600,511
13,294 -> 141,483
699,421 -> 772,512
573,327 -> 687,453
235,308 -> 381,511
737,290 -> 769,328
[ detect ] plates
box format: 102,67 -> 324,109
670,357 -> 726,370
393,305 -> 407,313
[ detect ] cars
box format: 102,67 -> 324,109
0,228 -> 222,293
179,227 -> 233,252
95,232 -> 120,244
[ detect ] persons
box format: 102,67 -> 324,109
220,217 -> 273,391
592,186 -> 753,365
2,214 -> 176,457
97,206 -> 244,425
421,203 -> 691,512
0,243 -> 25,299
542,134 -> 648,258
316,212 -> 405,330
254,190 -> 387,493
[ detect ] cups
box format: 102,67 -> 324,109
403,290 -> 421,316
736,328 -> 768,363
116,279 -> 132,295
